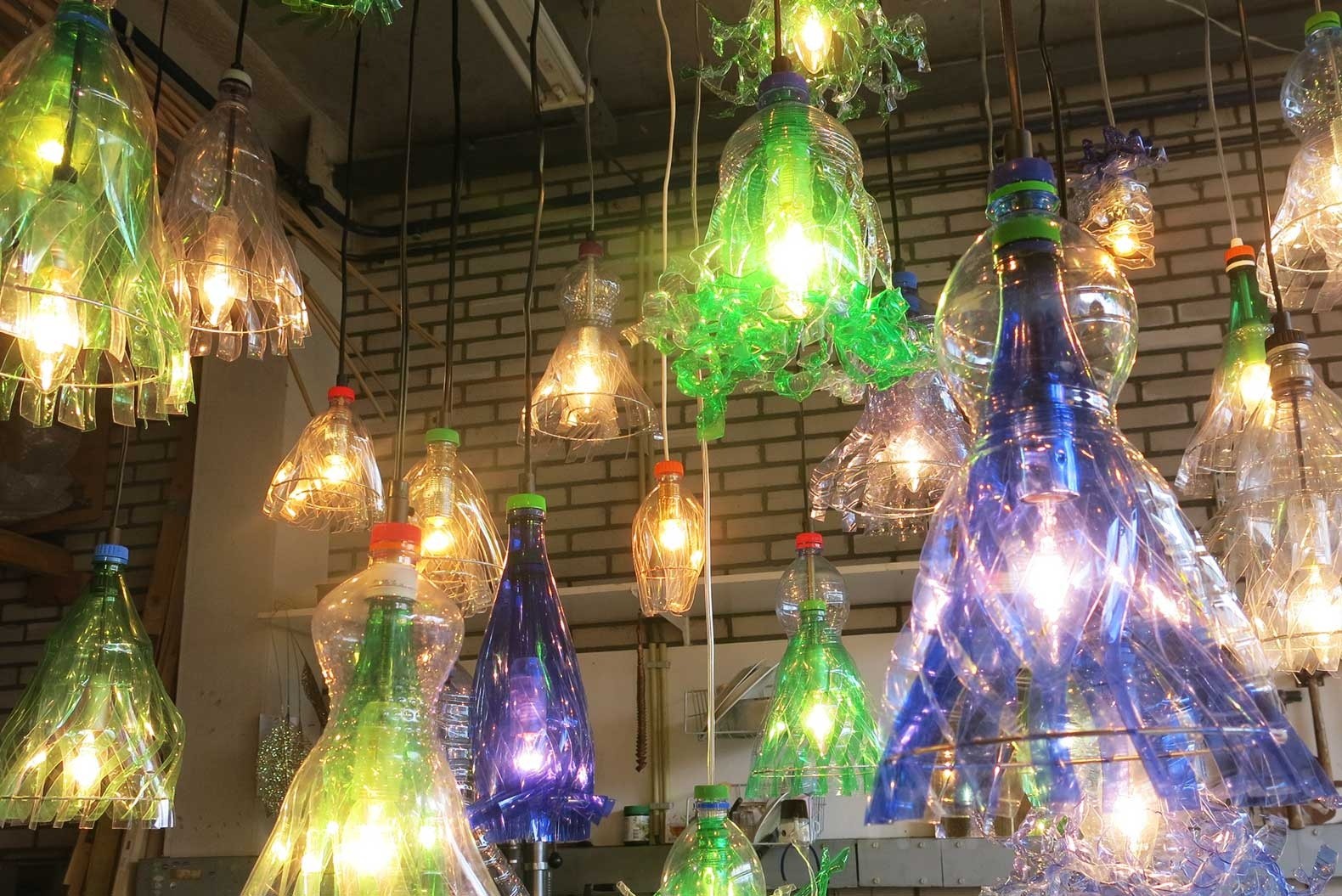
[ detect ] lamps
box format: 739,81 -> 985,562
0,0 -> 1342,896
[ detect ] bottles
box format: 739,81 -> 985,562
0,0 -> 1342,896
